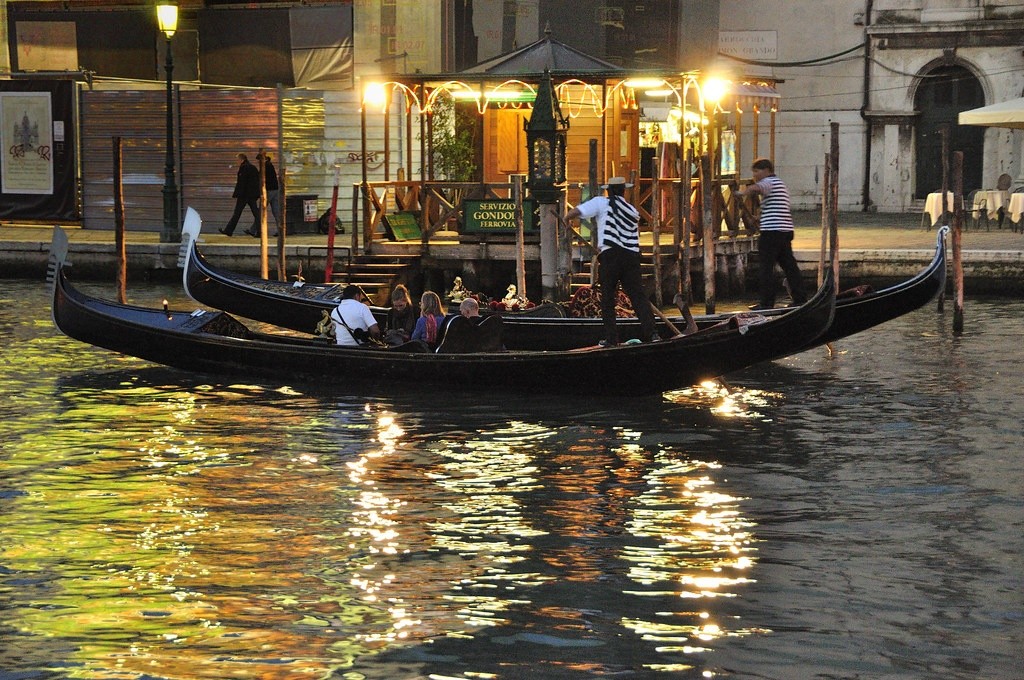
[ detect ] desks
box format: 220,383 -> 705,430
925,193 -> 965,232
972,191 -> 1011,229
1008,193 -> 1024,234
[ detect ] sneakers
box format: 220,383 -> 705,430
598,340 -> 619,348
649,336 -> 663,342
787,299 -> 808,307
748,302 -> 773,310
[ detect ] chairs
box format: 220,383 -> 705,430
964,190 -> 990,232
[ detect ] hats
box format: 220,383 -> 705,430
600,177 -> 634,189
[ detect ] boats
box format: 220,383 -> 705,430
45,219 -> 837,399
176,205 -> 951,362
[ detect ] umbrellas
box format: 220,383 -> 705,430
358,20 -> 783,256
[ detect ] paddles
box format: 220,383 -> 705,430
736,191 -> 795,299
550,206 -> 681,339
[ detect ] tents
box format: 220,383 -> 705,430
958,96 -> 1023,230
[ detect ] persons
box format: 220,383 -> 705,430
330,286 -> 485,345
734,160 -> 810,310
564,176 -> 662,347
217,152 -> 284,236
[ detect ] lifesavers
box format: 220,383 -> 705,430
689,187 -> 726,227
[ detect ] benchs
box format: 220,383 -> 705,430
426,314 -> 504,354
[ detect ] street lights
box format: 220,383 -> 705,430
155,4 -> 182,244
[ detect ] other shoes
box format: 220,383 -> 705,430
253,231 -> 260,238
273,232 -> 279,237
218,227 -> 233,236
242,229 -> 254,235
